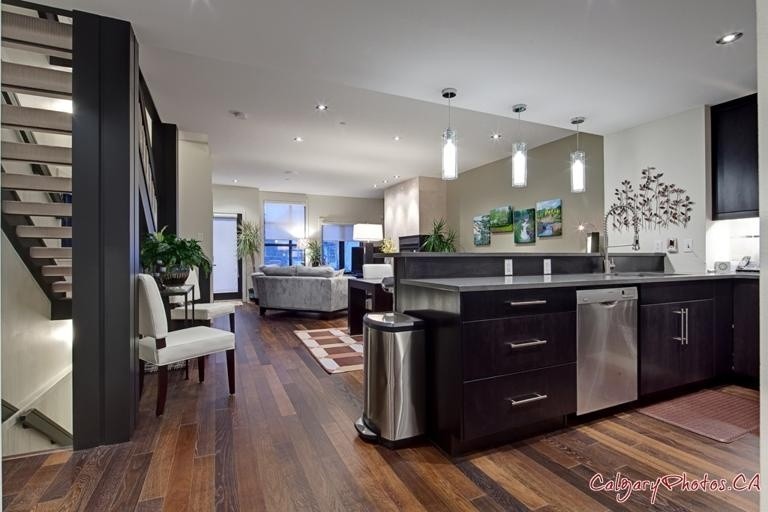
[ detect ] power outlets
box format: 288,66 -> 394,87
683,239 -> 692,252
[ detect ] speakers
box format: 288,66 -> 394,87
714,261 -> 731,274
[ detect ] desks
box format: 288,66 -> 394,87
169,285 -> 195,328
348,279 -> 392,336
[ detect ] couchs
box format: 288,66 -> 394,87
257,267 -> 356,319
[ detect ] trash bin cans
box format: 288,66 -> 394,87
354,311 -> 430,450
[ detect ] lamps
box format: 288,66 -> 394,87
440,89 -> 459,180
512,103 -> 529,189
353,223 -> 383,264
571,117 -> 586,192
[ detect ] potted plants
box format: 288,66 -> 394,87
234,220 -> 259,305
139,225 -> 212,286
307,241 -> 321,267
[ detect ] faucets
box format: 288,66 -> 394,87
601,204 -> 642,274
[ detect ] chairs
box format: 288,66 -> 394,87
137,273 -> 235,417
363,264 -> 395,313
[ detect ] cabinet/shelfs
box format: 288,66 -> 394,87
704,92 -> 759,222
424,288 -> 577,457
638,282 -> 713,403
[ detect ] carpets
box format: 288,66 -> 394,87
214,299 -> 243,306
636,388 -> 759,444
293,327 -> 364,374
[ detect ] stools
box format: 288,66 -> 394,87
170,303 -> 235,334
